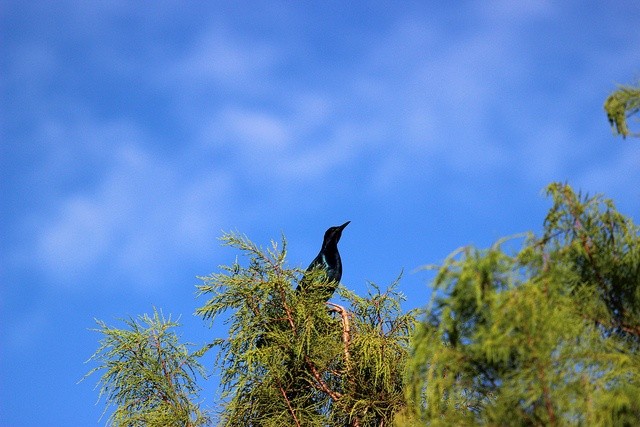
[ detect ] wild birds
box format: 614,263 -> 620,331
251,221 -> 350,363
